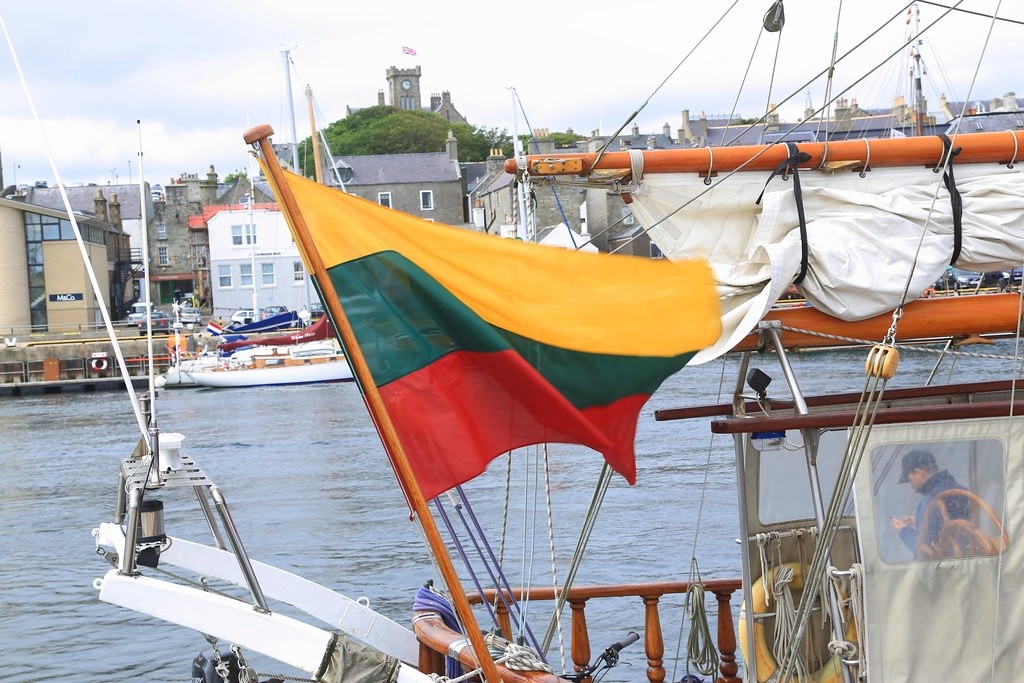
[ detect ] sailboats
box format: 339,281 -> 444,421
1,0 -> 1024,683
502,3 -> 1024,352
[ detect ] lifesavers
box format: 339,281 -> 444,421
739,562 -> 857,683
92,358 -> 108,371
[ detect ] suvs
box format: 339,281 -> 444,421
125,302 -> 157,326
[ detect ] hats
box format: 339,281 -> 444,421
897,449 -> 935,484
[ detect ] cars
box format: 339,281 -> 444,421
179,308 -> 203,325
137,309 -> 175,336
260,305 -> 289,321
302,302 -> 325,322
229,307 -> 265,326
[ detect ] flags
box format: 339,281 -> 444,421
255,153 -> 723,519
206,320 -> 223,336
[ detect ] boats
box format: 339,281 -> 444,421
180,309 -> 357,387
152,193 -> 316,390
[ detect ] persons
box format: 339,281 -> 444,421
890,449 -> 975,563
